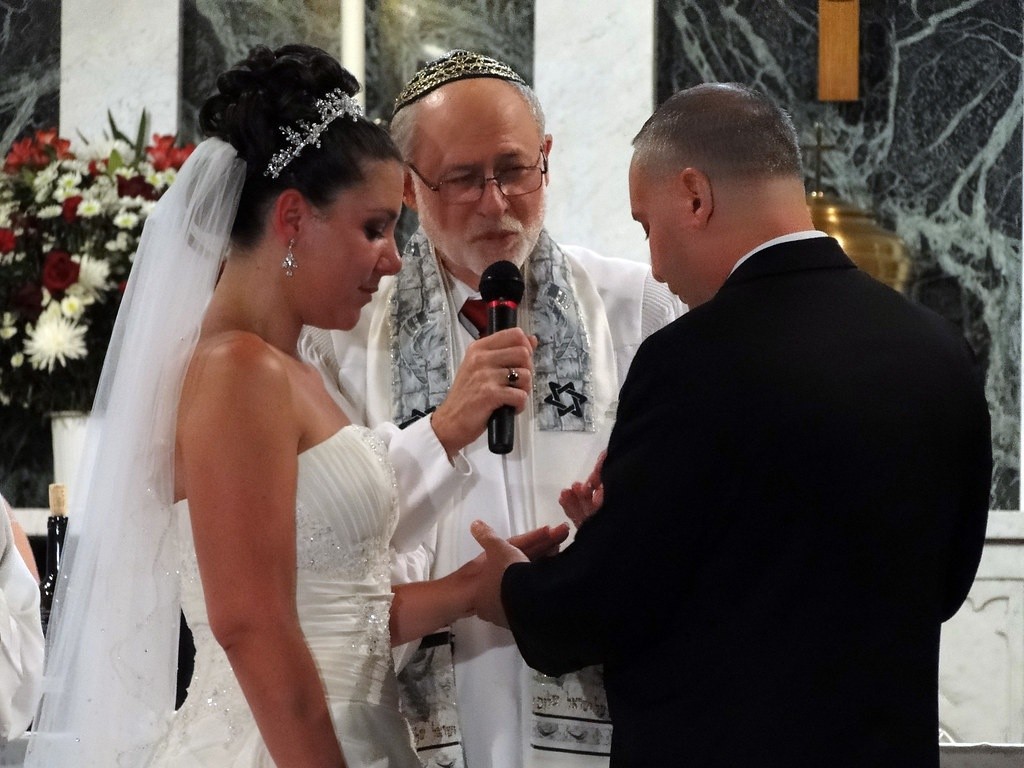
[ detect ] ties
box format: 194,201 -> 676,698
460,297 -> 493,339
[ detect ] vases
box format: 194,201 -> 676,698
50,410 -> 89,518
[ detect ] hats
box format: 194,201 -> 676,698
391,49 -> 528,116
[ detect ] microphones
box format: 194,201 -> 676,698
477,260 -> 526,453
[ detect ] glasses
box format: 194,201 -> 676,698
404,142 -> 549,205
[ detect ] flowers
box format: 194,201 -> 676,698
0,106 -> 194,410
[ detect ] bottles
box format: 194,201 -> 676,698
37,515 -> 67,629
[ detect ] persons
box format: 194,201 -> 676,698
0,42 -> 691,768
469,80 -> 997,768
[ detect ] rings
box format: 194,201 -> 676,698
507,368 -> 520,388
